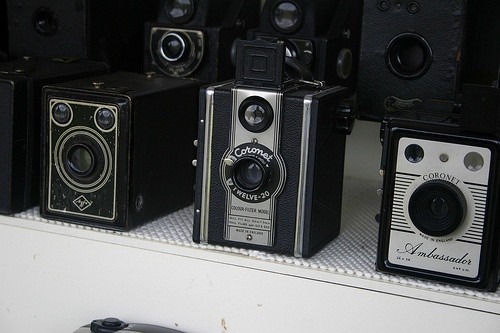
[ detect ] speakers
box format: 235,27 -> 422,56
1,1 -> 499,295
